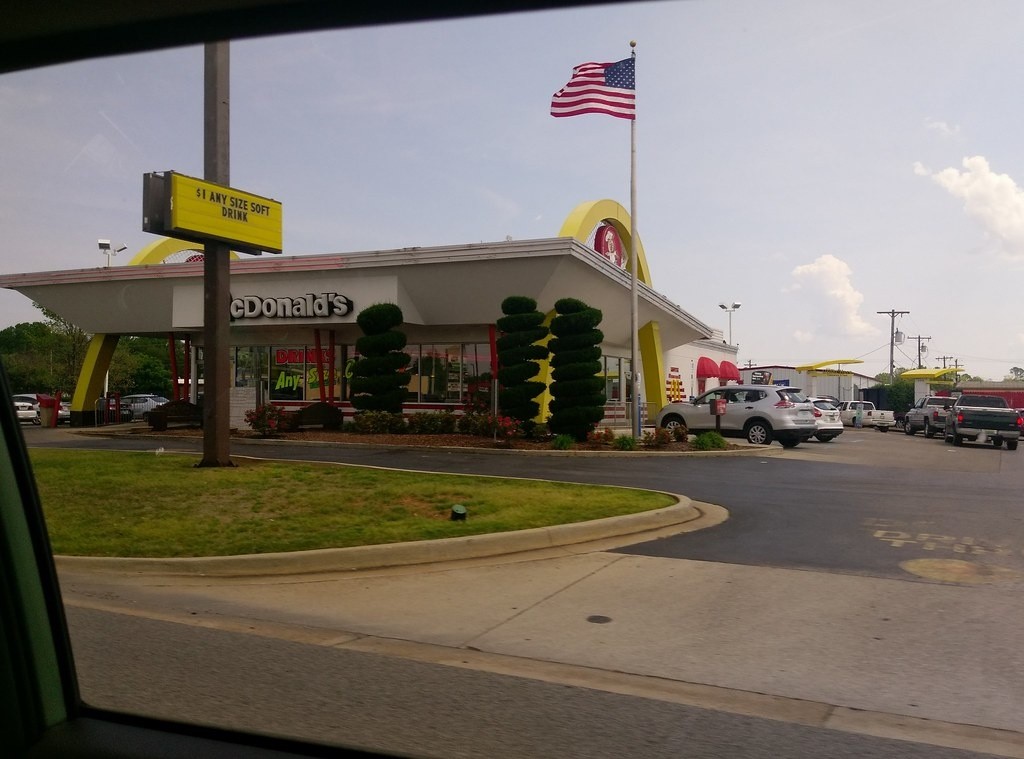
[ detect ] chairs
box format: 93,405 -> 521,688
730,391 -> 760,402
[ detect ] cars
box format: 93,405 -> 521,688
119,394 -> 171,422
1014,408 -> 1024,436
15,401 -> 37,423
12,393 -> 70,425
800,397 -> 845,443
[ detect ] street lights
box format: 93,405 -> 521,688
719,301 -> 741,345
98,239 -> 128,265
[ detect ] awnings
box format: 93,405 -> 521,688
719,361 -> 741,381
696,357 -> 721,379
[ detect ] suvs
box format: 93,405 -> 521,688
655,384 -> 819,446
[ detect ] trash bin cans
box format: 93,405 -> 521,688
35,393 -> 57,426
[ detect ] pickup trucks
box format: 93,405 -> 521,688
942,393 -> 1024,451
836,400 -> 898,433
903,395 -> 959,438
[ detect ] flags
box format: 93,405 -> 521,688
550,56 -> 636,120
